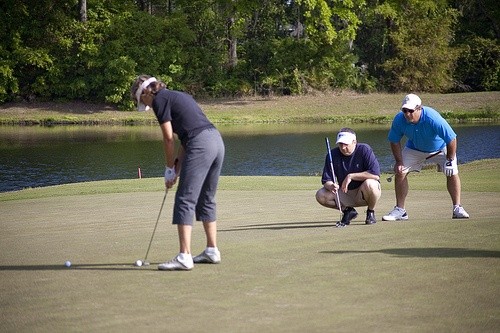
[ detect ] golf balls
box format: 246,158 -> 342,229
65,260 -> 71,267
136,260 -> 142,266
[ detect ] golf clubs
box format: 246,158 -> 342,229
387,150 -> 443,182
325,137 -> 343,221
142,158 -> 179,266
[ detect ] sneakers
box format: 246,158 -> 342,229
451,205 -> 469,217
365,211 -> 376,224
382,205 -> 408,221
156,253 -> 193,270
336,208 -> 358,228
192,248 -> 220,263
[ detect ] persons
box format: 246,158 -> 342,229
132,75 -> 225,271
382,94 -> 470,221
315,128 -> 381,228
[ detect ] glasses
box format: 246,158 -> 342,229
402,108 -> 419,114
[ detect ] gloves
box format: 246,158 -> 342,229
164,166 -> 176,188
443,160 -> 455,177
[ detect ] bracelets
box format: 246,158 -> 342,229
446,156 -> 454,161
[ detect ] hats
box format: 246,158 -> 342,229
400,94 -> 421,110
336,128 -> 356,144
135,77 -> 157,112
